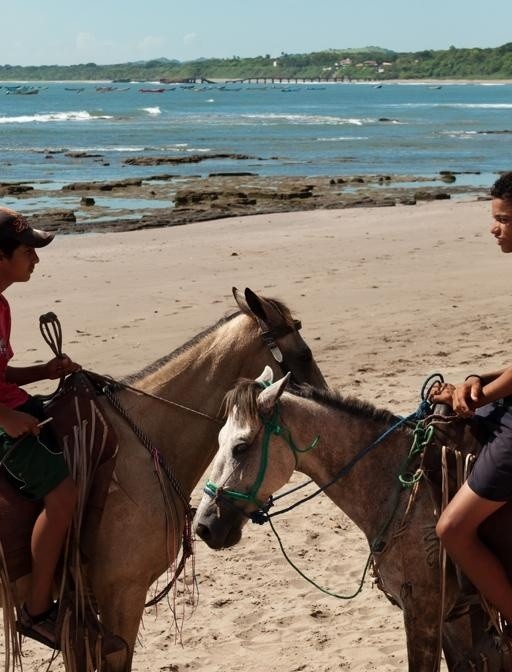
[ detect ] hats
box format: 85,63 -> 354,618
0,208 -> 56,248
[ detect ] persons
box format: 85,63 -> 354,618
429,171 -> 512,626
1,206 -> 83,653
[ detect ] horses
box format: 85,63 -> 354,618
0,286 -> 333,672
188,363 -> 512,672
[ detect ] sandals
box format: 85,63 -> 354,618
14,603 -> 63,650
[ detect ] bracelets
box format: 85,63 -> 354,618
465,375 -> 484,383
